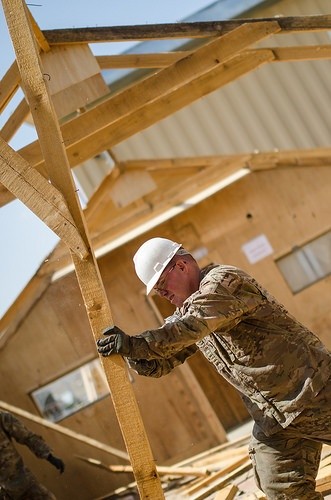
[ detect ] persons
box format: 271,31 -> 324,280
97,236 -> 331,500
0,408 -> 65,500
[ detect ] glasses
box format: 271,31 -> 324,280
155,260 -> 187,293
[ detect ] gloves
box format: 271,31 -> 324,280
97,326 -> 150,360
123,351 -> 157,375
46,455 -> 65,475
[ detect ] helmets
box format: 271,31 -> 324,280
133,236 -> 183,296
